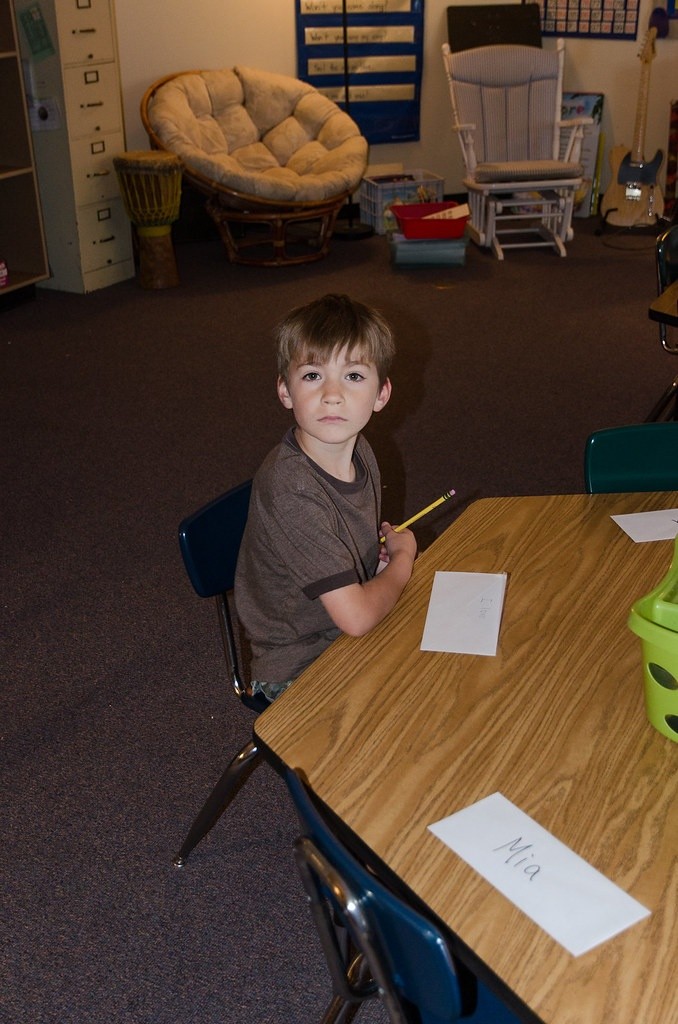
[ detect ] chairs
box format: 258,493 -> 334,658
650,224 -> 678,353
586,421 -> 677,492
172,480 -> 273,867
286,767 -> 528,1023
141,64 -> 367,267
445,37 -> 593,259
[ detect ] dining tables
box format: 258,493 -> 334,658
388,233 -> 465,267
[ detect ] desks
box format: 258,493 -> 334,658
256,492 -> 678,1023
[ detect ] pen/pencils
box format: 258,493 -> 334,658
379,489 -> 456,543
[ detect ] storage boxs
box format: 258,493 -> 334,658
359,169 -> 445,233
629,533 -> 678,748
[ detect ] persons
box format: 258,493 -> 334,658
229,294 -> 413,1003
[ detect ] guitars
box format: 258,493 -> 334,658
599,26 -> 664,234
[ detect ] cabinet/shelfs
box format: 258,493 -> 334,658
0,0 -> 49,317
11,0 -> 135,293
